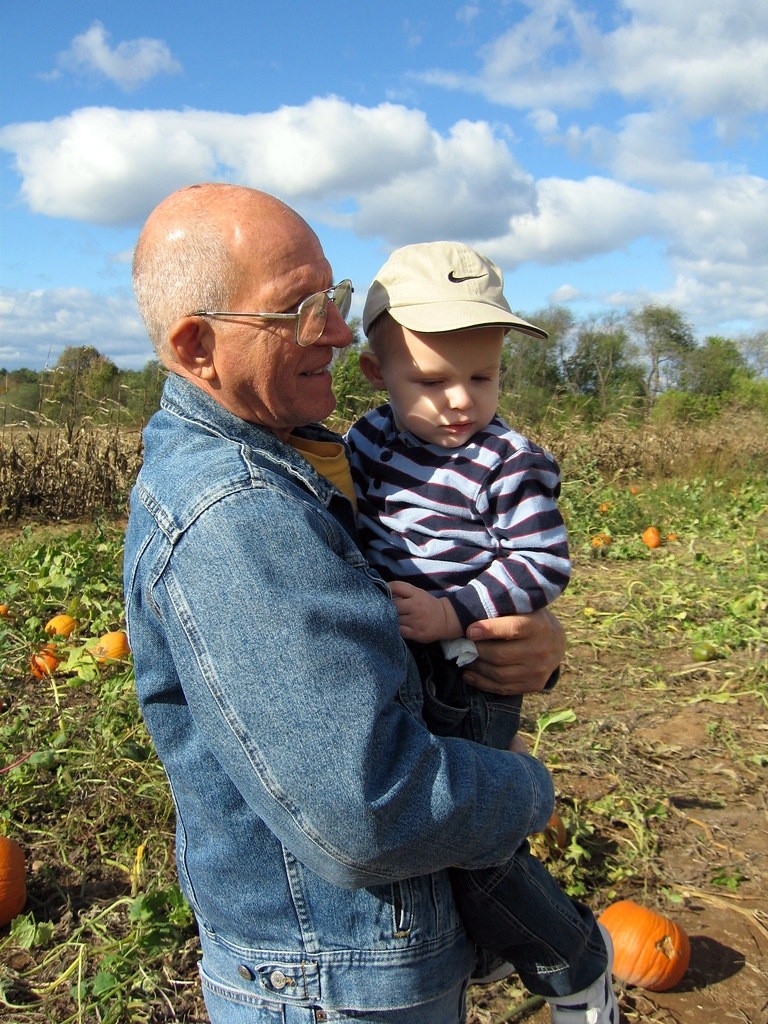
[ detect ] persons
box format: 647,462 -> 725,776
124,186 -> 564,1024
341,241 -> 618,1024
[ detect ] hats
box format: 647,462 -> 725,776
362,240 -> 552,343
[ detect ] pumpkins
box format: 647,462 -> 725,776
586,485 -> 661,549
0,835 -> 27,931
595,901 -> 691,991
0,605 -> 130,679
530,811 -> 567,848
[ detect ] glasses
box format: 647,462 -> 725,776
189,277 -> 354,349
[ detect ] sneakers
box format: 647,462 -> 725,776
544,923 -> 619,1024
464,963 -> 516,989
